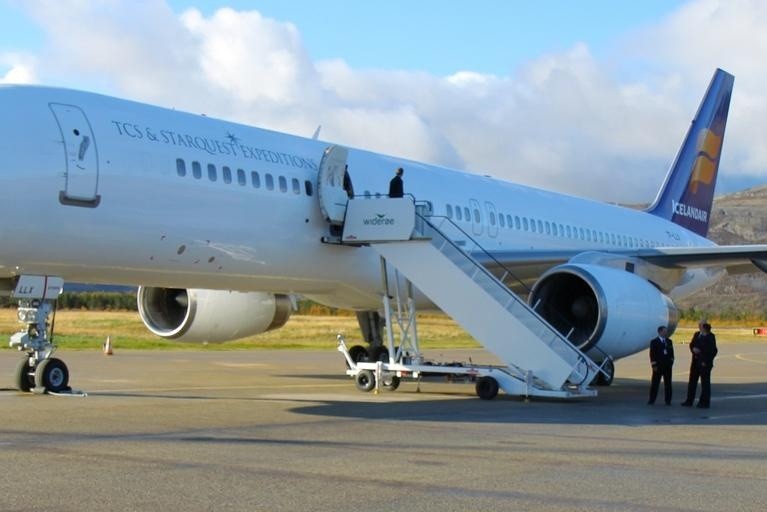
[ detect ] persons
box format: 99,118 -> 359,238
696,324 -> 715,409
647,326 -> 675,405
681,319 -> 718,406
389,167 -> 404,197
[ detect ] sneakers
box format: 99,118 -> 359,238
648,400 -> 710,409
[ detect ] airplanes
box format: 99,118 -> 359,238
0,61 -> 766,406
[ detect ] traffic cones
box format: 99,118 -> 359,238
104,335 -> 115,356
